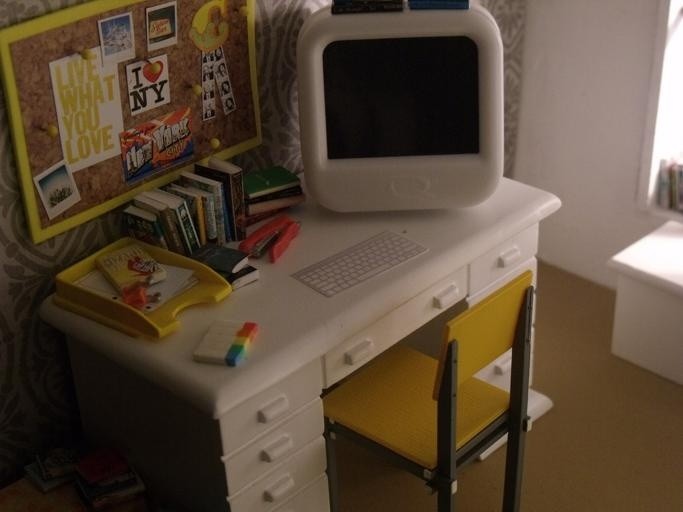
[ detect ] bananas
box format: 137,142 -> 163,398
290,232 -> 430,298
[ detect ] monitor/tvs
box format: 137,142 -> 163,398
659,159 -> 683,214
124,156 -> 306,291
96,242 -> 168,295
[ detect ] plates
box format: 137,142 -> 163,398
604,219 -> 683,383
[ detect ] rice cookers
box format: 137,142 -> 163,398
237,214 -> 298,263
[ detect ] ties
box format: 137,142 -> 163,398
298,2 -> 509,214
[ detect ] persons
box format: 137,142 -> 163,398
198,44 -> 236,121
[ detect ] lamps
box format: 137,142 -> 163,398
323,267 -> 533,512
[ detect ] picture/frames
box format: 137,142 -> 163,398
38,173 -> 563,511
